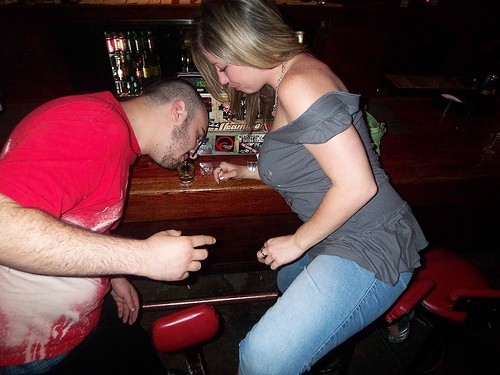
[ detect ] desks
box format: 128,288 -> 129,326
0,93 -> 500,280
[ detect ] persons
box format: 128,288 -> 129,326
0,81 -> 218,375
191,0 -> 429,375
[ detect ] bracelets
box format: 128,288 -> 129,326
247,161 -> 257,173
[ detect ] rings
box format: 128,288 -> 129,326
218,166 -> 221,171
260,249 -> 266,256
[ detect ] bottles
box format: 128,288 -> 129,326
104,31 -> 159,96
166,30 -> 197,72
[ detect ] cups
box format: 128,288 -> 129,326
483,133 -> 500,153
437,93 -> 463,114
483,75 -> 499,95
176,161 -> 195,188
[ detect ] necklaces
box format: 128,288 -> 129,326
270,61 -> 288,116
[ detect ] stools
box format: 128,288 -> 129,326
412,248 -> 489,323
